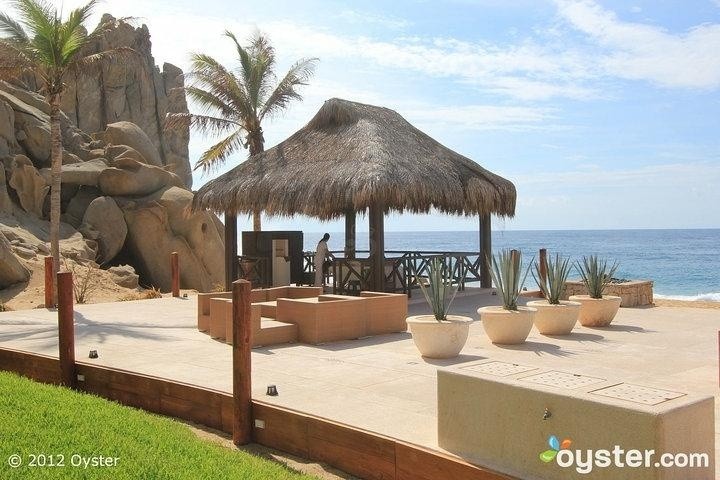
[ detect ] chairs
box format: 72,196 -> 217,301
198,249 -> 409,347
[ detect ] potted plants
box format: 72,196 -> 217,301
527,250 -> 581,337
569,254 -> 627,329
406,261 -> 475,359
477,247 -> 536,346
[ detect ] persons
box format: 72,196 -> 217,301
313,233 -> 336,293
